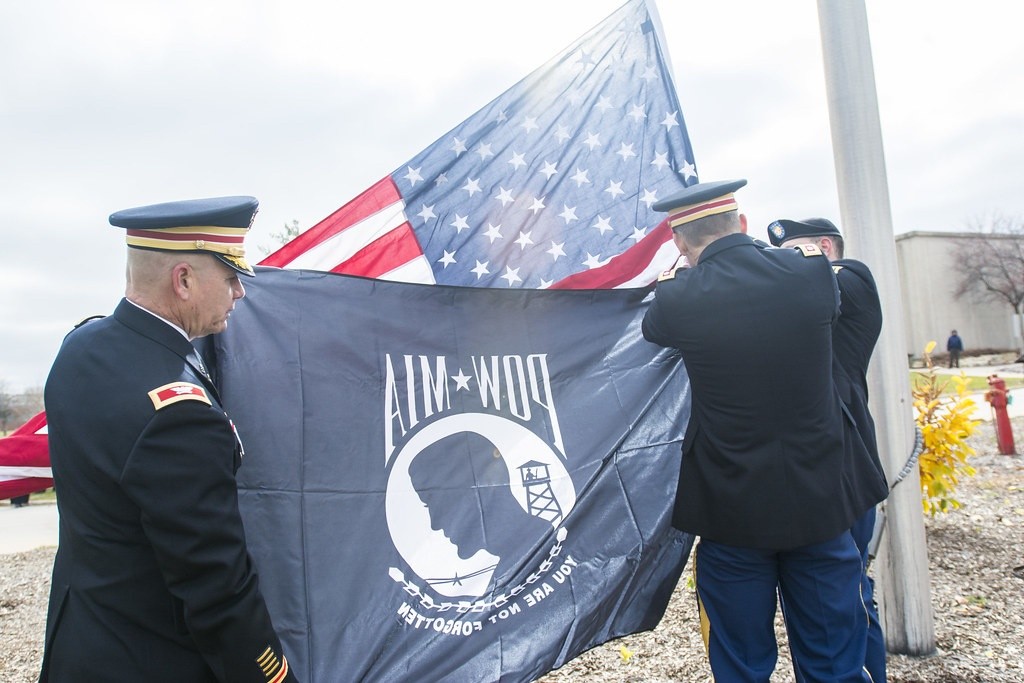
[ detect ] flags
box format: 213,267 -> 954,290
0,0 -> 700,502
193,265 -> 695,682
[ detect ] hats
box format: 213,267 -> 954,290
652,179 -> 747,228
108,196 -> 259,277
767,217 -> 843,247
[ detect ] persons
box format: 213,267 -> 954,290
766,218 -> 887,683
947,330 -> 963,368
640,178 -> 890,683
37,194 -> 300,683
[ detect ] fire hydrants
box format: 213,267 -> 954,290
985,375 -> 1017,456
